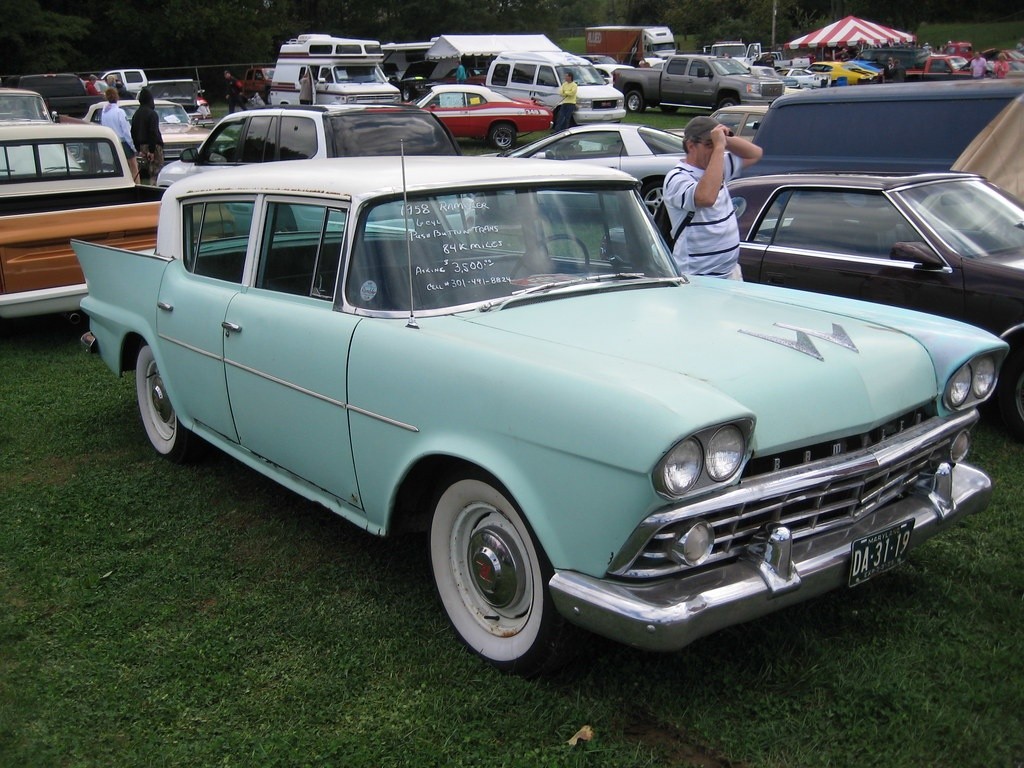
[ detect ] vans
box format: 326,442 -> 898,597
486,51 -> 626,129
98,69 -> 148,93
851,47 -> 929,71
740,79 -> 1024,179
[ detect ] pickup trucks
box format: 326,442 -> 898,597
236,68 -> 275,104
614,55 -> 786,114
905,42 -> 997,80
17,75 -> 102,120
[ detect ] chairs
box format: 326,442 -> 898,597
857,205 -> 914,260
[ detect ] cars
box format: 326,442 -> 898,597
988,47 -> 1024,74
578,55 -> 634,85
83,79 -> 235,167
0,86 -> 237,324
385,60 -> 439,104
747,60 -> 886,89
70,156 -> 1023,679
408,83 -> 767,224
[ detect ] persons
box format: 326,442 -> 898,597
224,71 -> 248,114
299,72 -> 318,105
131,88 -> 165,186
805,38 -> 1024,84
663,116 -> 763,281
101,87 -> 141,185
455,61 -> 467,84
551,72 -> 577,133
752,52 -> 776,69
85,75 -> 128,100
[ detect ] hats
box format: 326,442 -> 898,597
684,116 -> 734,138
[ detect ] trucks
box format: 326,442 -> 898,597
703,40 -> 762,69
586,26 -> 681,62
268,33 -> 401,109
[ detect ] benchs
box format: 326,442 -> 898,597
200,233 -> 528,315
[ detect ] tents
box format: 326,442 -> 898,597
784,15 -> 916,62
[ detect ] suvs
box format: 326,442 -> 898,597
155,103 -> 461,188
